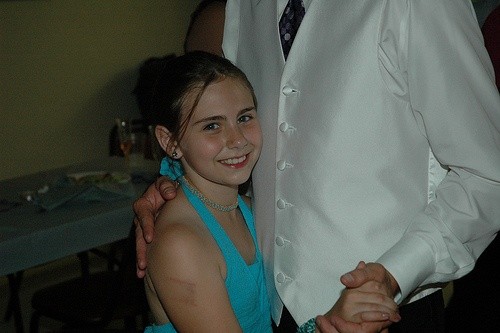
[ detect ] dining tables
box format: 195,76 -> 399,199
0,155 -> 151,333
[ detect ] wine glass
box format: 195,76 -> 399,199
118,118 -> 133,172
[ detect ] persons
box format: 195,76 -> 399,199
141,46 -> 403,332
127,0 -> 500,332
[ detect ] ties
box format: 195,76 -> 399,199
278,0 -> 307,60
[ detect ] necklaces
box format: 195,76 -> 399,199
181,174 -> 240,212
